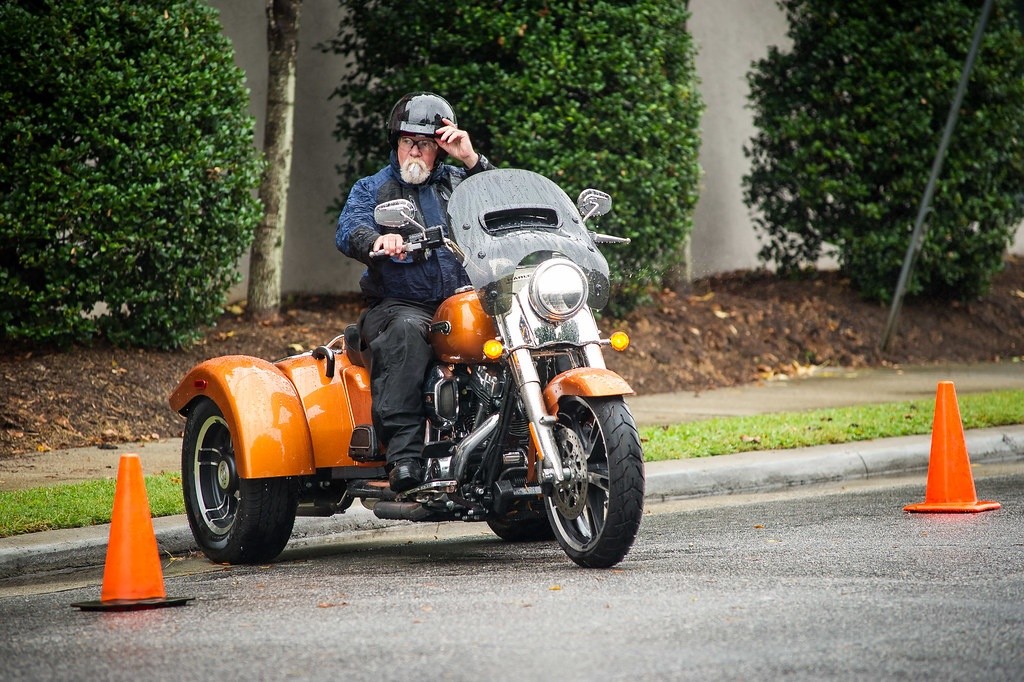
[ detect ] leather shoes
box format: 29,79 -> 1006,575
388,460 -> 425,493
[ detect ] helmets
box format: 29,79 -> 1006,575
385,91 -> 457,136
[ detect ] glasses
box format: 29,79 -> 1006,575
397,137 -> 440,152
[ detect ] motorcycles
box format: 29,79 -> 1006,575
171,168 -> 649,572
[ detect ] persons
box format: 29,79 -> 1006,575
335,90 -> 500,492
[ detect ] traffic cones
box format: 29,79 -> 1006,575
901,381 -> 1002,512
69,455 -> 198,612
